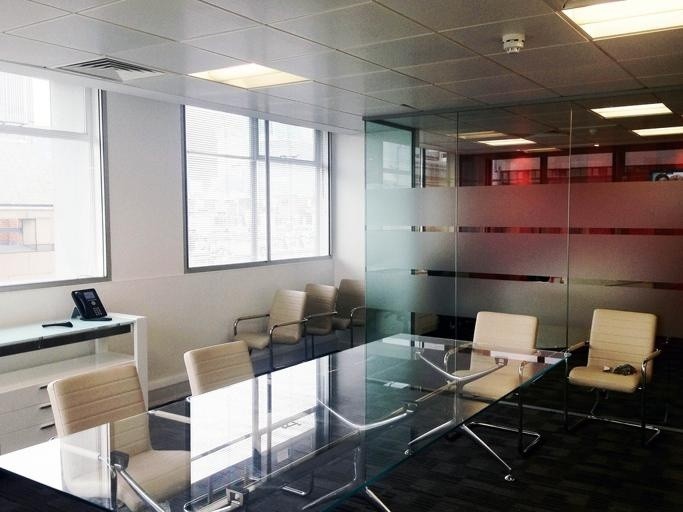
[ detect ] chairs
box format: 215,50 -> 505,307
441,305 -> 667,457
184,341 -> 317,506
230,277 -> 367,370
46,363 -> 207,511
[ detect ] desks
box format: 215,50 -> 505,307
0,330 -> 572,511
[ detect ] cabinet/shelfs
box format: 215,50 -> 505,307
0,312 -> 149,457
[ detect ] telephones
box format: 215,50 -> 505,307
71,288 -> 108,319
